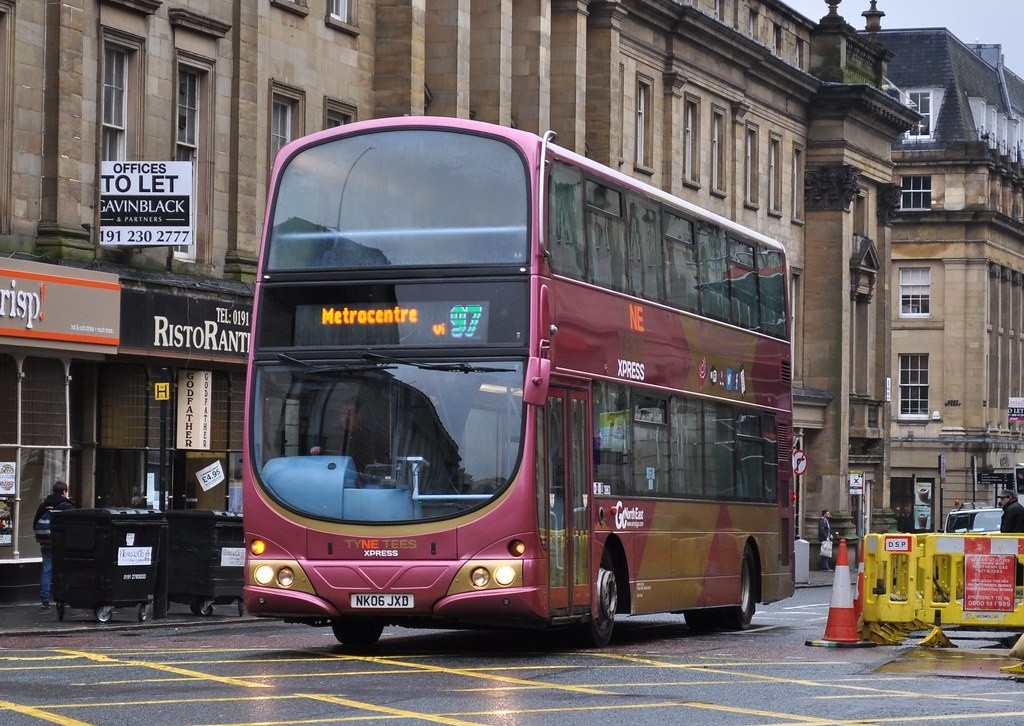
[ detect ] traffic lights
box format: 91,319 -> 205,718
1015,465 -> 1024,494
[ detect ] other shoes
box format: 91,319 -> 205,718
42,601 -> 52,608
64,603 -> 69,607
823,568 -> 834,572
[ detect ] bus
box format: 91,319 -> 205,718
242,112 -> 797,652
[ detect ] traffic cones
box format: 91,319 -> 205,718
804,538 -> 878,649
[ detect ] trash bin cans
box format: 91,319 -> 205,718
49,507 -> 169,624
164,509 -> 246,617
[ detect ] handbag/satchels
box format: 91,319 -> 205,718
819,537 -> 833,557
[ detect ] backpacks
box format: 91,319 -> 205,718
34,502 -> 70,549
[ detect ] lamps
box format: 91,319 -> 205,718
980,132 -> 990,141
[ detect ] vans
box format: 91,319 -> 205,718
944,506 -> 1006,537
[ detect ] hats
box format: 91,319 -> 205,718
997,490 -> 1013,497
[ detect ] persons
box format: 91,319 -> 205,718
818,510 -> 838,571
310,399 -> 389,474
33,482 -> 73,609
997,490 -> 1024,586
